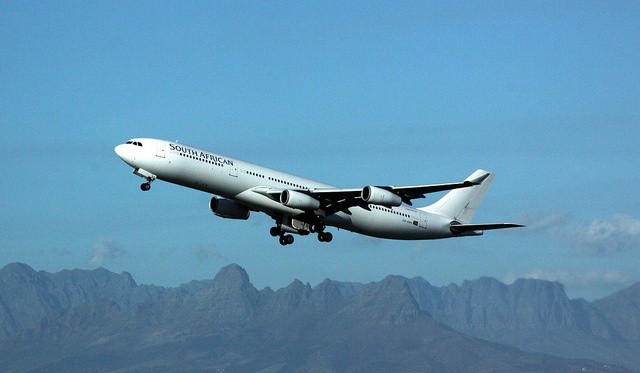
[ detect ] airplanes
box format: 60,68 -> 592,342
113,137 -> 526,246
